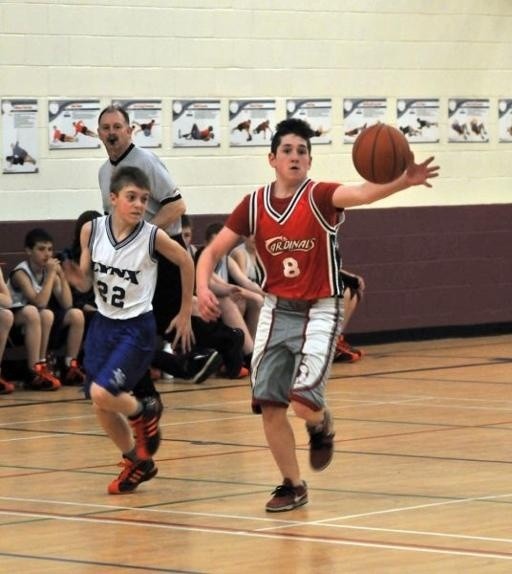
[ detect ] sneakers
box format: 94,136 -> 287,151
265,478 -> 309,513
216,359 -> 249,379
1,376 -> 15,393
58,358 -> 87,385
309,405 -> 334,473
334,335 -> 365,363
184,348 -> 221,382
19,359 -> 63,392
132,392 -> 164,459
107,448 -> 157,495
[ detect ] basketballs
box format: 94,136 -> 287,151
353,123 -> 410,184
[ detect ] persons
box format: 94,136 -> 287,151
58,164 -> 196,496
47,211 -> 161,381
2,272 -> 16,396
193,116 -> 441,514
95,104 -> 250,421
3,227 -> 86,391
332,268 -> 365,364
5,115 -> 488,169
161,214 -> 266,379
